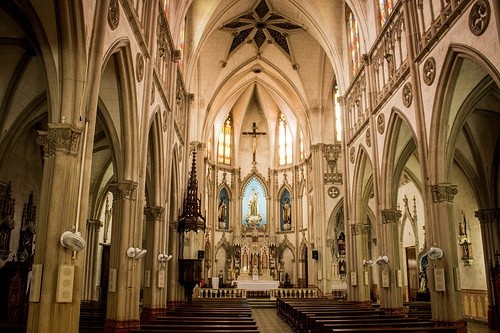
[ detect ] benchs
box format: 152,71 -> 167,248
276,296 -> 467,333
80,298 -> 259,333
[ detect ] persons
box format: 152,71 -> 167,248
418,267 -> 429,293
338,259 -> 347,274
218,198 -> 227,221
285,273 -> 290,282
282,200 -> 291,224
246,133 -> 262,160
199,279 -> 205,289
248,189 -> 258,216
219,269 -> 224,286
338,232 -> 345,240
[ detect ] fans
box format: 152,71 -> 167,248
60,231 -> 86,252
126,247 -> 149,259
159,253 -> 172,262
428,246 -> 444,259
376,255 -> 388,265
363,260 -> 376,266
